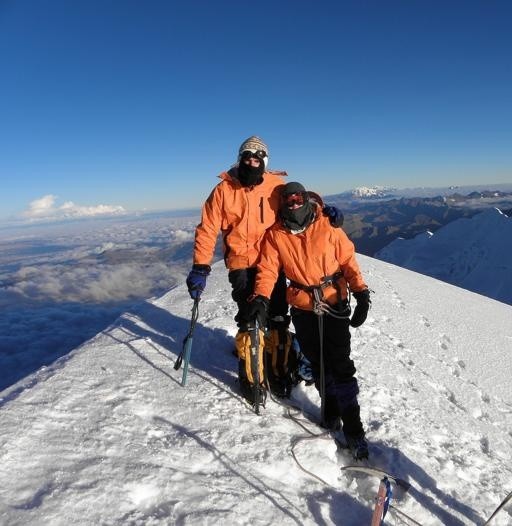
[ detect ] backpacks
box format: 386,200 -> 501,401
289,333 -> 315,386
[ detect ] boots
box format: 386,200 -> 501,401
239,359 -> 264,403
265,351 -> 292,399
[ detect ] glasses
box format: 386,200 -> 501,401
284,192 -> 305,207
240,150 -> 267,160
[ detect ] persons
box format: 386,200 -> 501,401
185,135 -> 346,405
248,180 -> 371,461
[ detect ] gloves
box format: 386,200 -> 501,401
245,295 -> 270,321
322,203 -> 344,228
350,290 -> 371,328
186,266 -> 208,299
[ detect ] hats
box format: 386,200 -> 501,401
280,182 -> 313,230
238,136 -> 269,170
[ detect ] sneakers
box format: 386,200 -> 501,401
343,425 -> 368,458
321,409 -> 340,430
